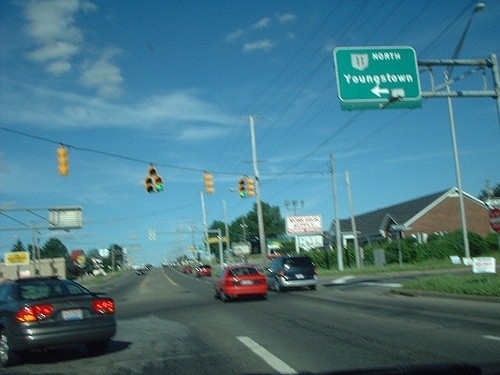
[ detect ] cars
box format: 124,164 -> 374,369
213,264 -> 269,302
136,269 -> 144,275
196,265 -> 212,277
183,266 -> 193,274
0,275 -> 117,368
264,256 -> 318,292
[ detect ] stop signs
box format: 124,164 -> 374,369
489,208 -> 500,233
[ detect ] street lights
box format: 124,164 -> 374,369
442,3 -> 487,261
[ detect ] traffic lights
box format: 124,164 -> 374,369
145,167 -> 164,193
239,179 -> 247,198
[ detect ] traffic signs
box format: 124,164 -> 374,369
332,46 -> 423,102
